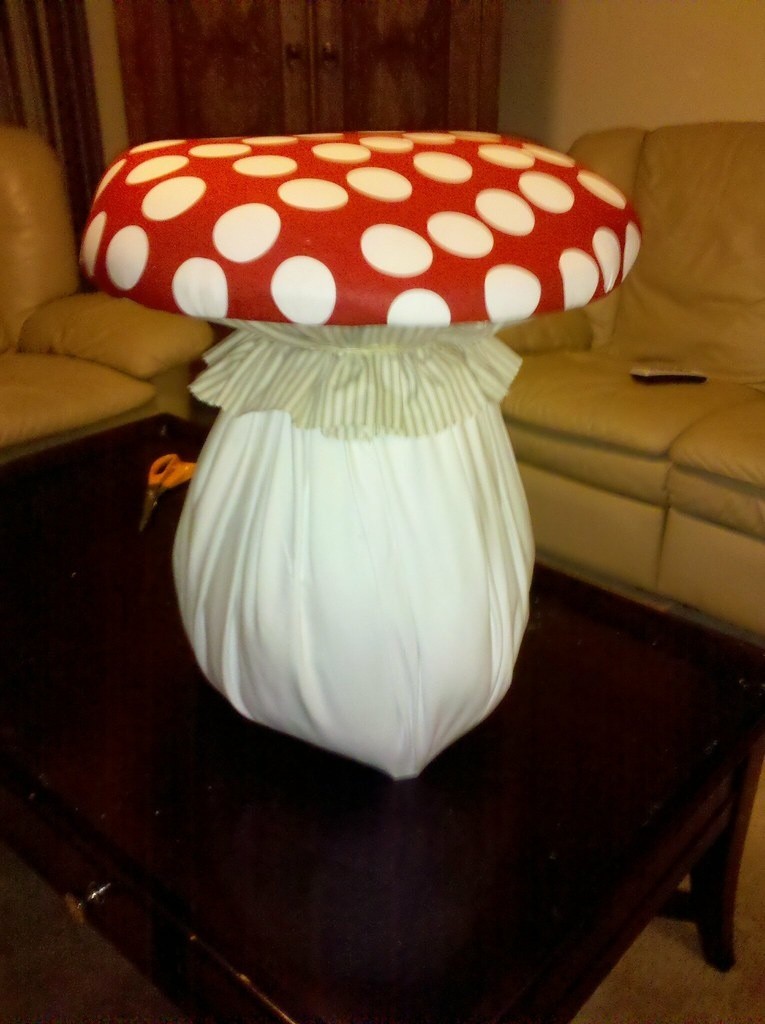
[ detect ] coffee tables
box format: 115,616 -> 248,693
0,413 -> 765,1024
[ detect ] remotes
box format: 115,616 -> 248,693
628,367 -> 709,384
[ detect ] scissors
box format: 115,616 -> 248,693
136,452 -> 196,537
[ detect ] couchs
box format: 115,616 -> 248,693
498,122 -> 765,644
0,128 -> 215,466
